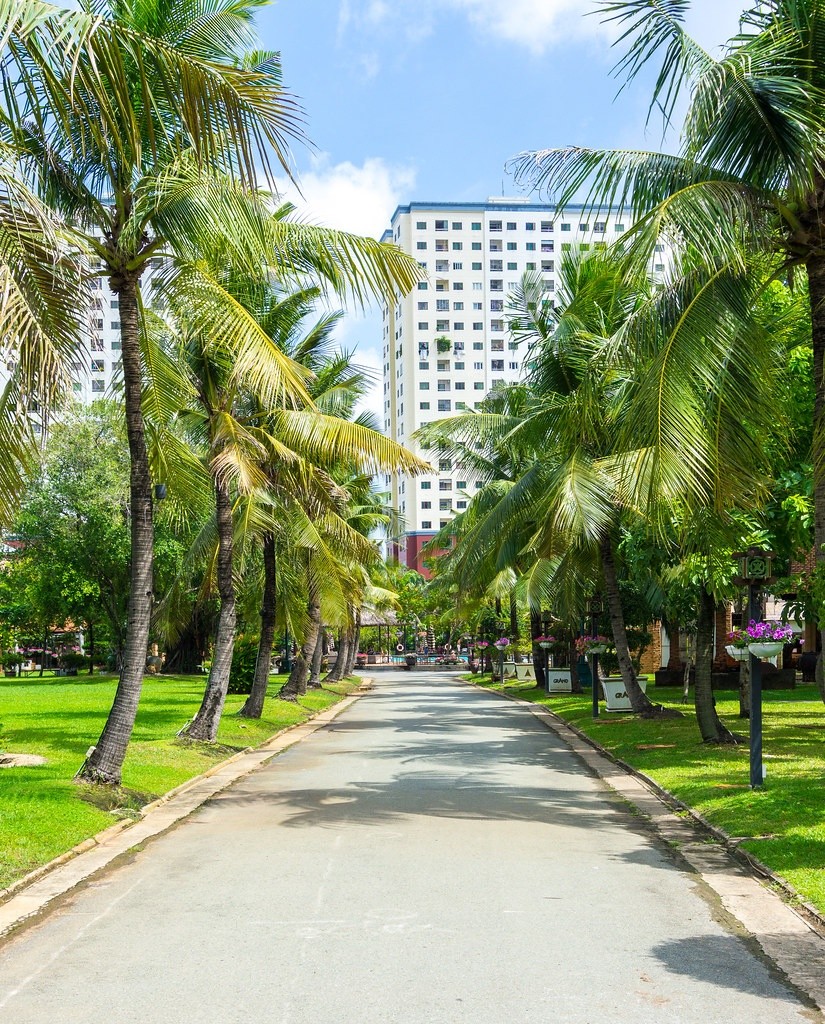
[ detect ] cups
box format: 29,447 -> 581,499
762,764 -> 767,778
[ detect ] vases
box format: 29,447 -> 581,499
749,642 -> 783,658
478,645 -> 486,650
540,641 -> 553,648
725,645 -> 750,659
357,658 -> 367,666
497,645 -> 506,650
406,659 -> 417,666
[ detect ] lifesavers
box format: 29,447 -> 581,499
445,644 -> 451,650
397,644 -> 404,651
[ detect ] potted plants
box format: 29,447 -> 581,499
492,579 -> 662,713
0,653 -> 29,677
56,653 -> 92,676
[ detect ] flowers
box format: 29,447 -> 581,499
494,638 -> 511,646
404,652 -> 418,658
534,634 -> 557,643
745,619 -> 805,644
729,625 -> 747,649
575,636 -> 613,661
356,653 -> 367,659
476,641 -> 488,646
